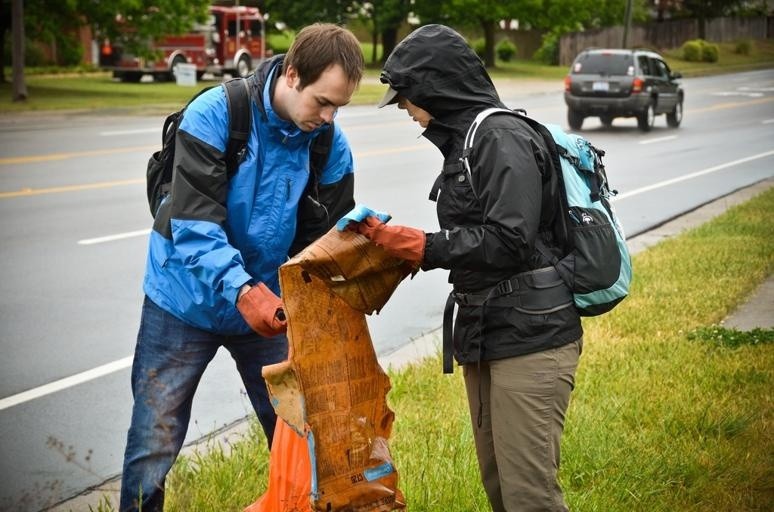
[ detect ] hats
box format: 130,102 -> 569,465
378,87 -> 397,110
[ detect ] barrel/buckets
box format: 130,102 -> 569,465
175,63 -> 198,88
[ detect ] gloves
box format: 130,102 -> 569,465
360,215 -> 427,271
237,281 -> 287,340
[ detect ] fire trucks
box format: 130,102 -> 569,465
97,3 -> 272,83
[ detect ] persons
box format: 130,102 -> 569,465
115,23 -> 363,510
359,25 -> 587,511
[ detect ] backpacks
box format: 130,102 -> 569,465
461,107 -> 632,318
147,74 -> 334,220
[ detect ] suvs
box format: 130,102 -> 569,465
563,46 -> 684,131
